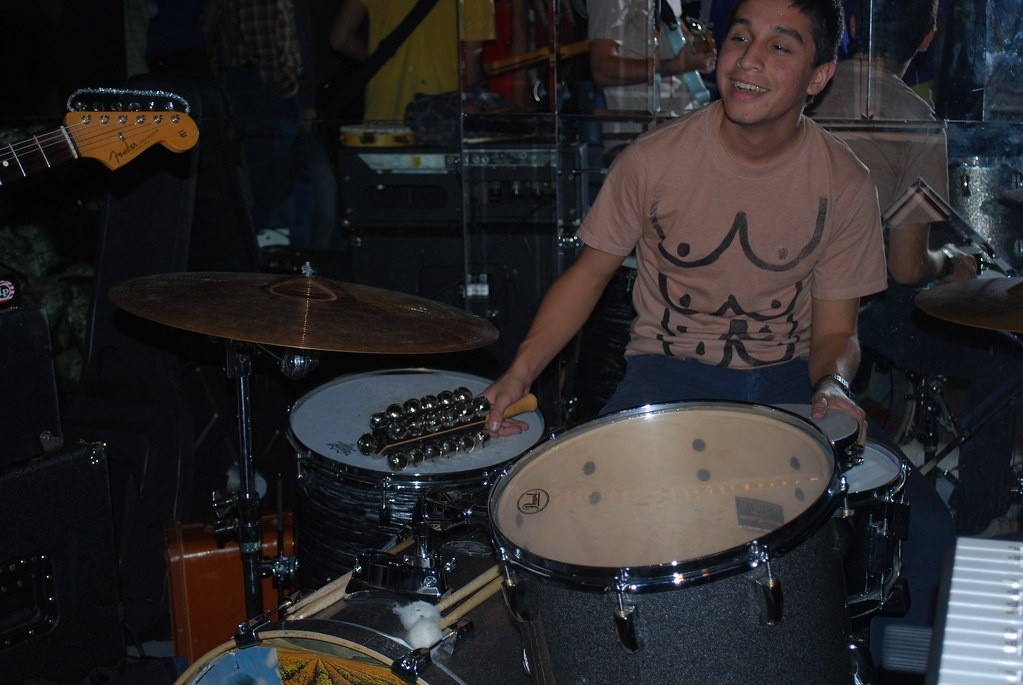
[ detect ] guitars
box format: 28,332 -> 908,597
1,85 -> 201,184
679,13 -> 717,75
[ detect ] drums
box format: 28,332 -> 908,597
488,404 -> 876,685
286,368 -> 548,593
947,152 -> 1022,271
174,589 -> 541,685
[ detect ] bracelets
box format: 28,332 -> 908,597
812,373 -> 851,399
936,248 -> 954,280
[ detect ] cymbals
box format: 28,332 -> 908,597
111,270 -> 501,356
914,278 -> 1023,333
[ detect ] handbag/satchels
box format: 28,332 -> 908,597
314,52 -> 368,152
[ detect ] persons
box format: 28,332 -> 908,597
475,0 -> 954,627
806,2 -> 1022,538
122,1 -> 718,168
